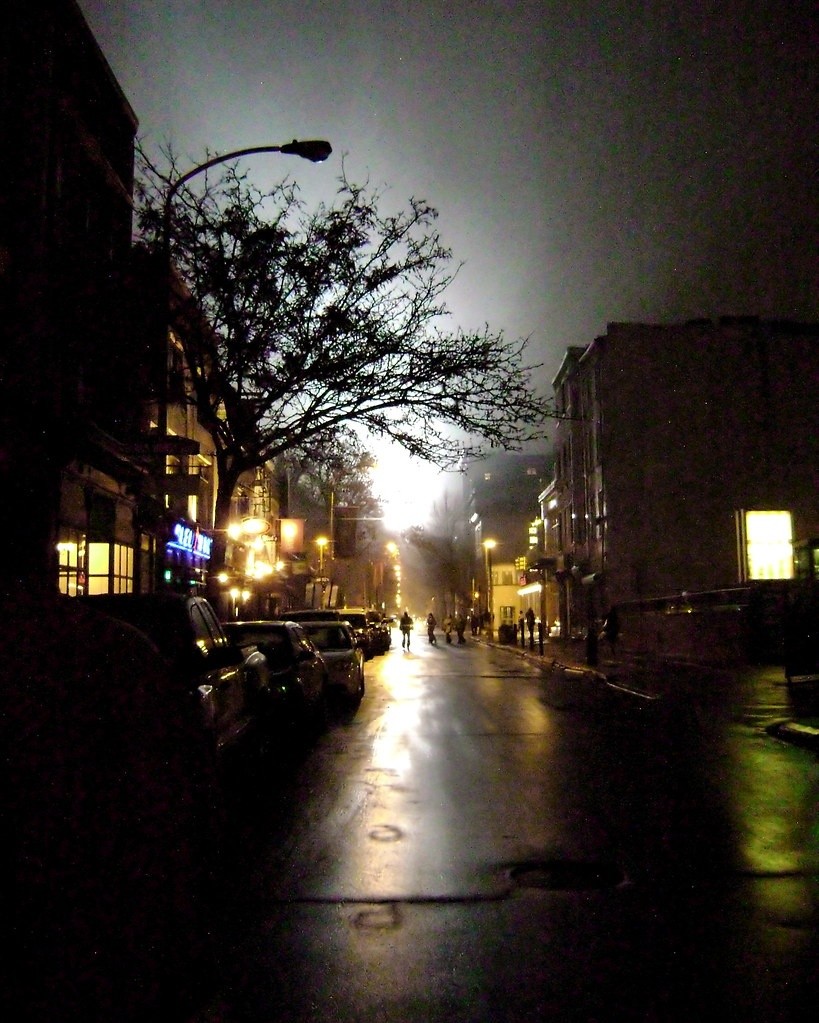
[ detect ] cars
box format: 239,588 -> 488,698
216,620 -> 324,738
295,619 -> 370,713
280,607 -> 394,661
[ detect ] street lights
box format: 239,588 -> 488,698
156,140 -> 333,591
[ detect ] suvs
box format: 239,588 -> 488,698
75,589 -> 254,753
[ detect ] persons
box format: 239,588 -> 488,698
598,609 -> 622,667
426,613 -> 437,644
443,613 -> 467,645
518,607 -> 535,644
471,612 -> 484,636
399,611 -> 414,651
